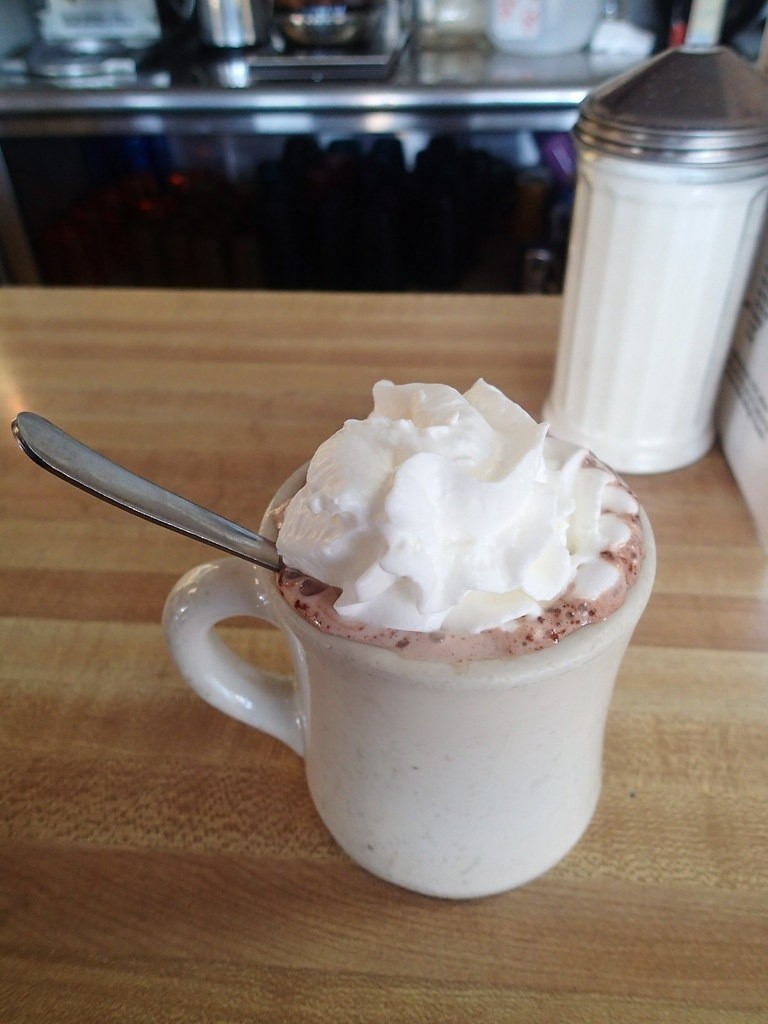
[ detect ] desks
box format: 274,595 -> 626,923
0,44 -> 653,288
0,287 -> 768,1024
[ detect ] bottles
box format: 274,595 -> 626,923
541,43 -> 767,473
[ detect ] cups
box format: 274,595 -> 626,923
161,463 -> 658,899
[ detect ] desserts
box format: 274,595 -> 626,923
277,379 -> 642,635
